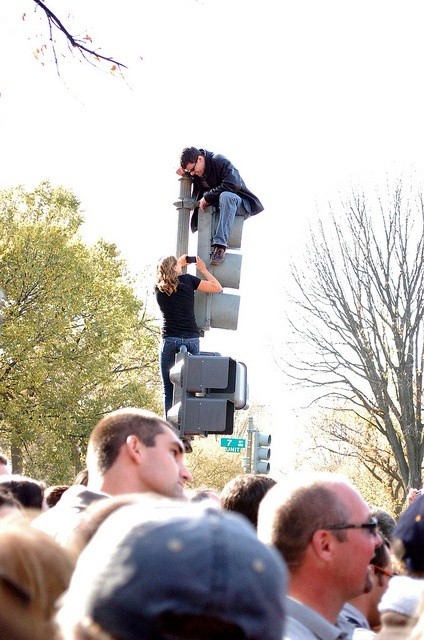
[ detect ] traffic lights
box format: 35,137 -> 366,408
167,346 -> 236,438
253,430 -> 271,474
194,205 -> 244,333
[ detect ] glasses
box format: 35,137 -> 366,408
320,517 -> 378,538
185,164 -> 196,175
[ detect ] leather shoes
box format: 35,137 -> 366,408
211,246 -> 225,265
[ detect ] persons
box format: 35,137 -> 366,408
371,510 -> 401,571
336,535 -> 393,640
220,474 -> 278,531
45,486 -> 67,507
1,517 -> 75,640
190,489 -> 221,504
408,488 -> 424,505
0,496 -> 25,522
178,147 -> 263,264
378,491 -> 424,640
154,255 -> 223,454
28,408 -> 194,550
258,472 -> 382,640
0,456 -> 13,477
0,477 -> 43,508
55,503 -> 290,640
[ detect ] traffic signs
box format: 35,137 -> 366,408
221,438 -> 246,452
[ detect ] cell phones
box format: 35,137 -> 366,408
186,256 -> 198,263
